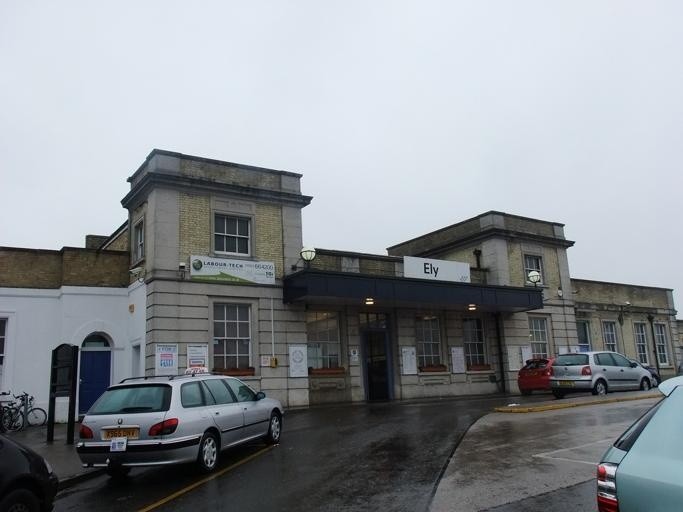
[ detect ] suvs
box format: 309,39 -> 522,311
596,375 -> 682,512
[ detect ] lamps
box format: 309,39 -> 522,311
291,246 -> 317,271
528,270 -> 540,288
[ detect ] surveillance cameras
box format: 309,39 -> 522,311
129,266 -> 142,275
625,301 -> 631,306
178,261 -> 187,271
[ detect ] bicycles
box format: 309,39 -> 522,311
0,390 -> 47,433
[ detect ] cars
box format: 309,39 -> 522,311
0,434 -> 58,512
75,373 -> 285,474
517,357 -> 554,394
629,358 -> 661,389
548,351 -> 660,400
